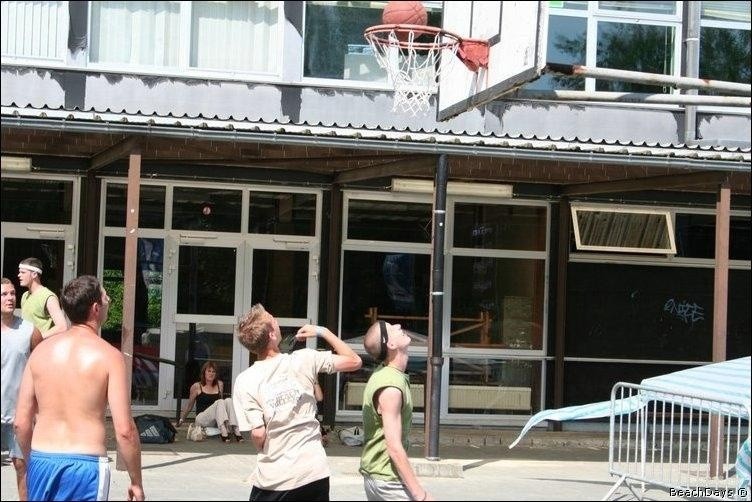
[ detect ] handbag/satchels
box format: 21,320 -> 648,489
186,423 -> 207,441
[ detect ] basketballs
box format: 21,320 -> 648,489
382,1 -> 427,42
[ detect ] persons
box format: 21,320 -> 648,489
179,303 -> 363,502
359,320 -> 429,501
1,258 -> 145,501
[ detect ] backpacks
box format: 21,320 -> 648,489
132,414 -> 177,444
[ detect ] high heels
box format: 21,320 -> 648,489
220,431 -> 244,443
